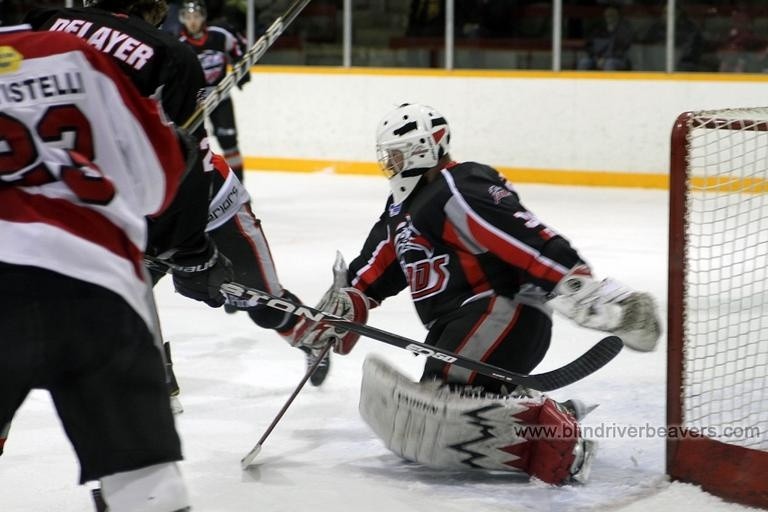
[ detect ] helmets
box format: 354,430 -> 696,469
63,0 -> 169,29
376,104 -> 450,206
178,0 -> 207,23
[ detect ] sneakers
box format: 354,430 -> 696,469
306,345 -> 330,386
553,400 -> 585,474
164,342 -> 179,397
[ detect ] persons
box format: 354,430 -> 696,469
0,3 -> 237,512
305,104 -> 662,489
176,1 -> 248,185
142,142 -> 330,387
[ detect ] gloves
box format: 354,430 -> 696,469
292,286 -> 381,355
236,71 -> 250,89
170,234 -> 233,307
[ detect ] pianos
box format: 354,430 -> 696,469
144,256 -> 623,392
241,338 -> 337,470
